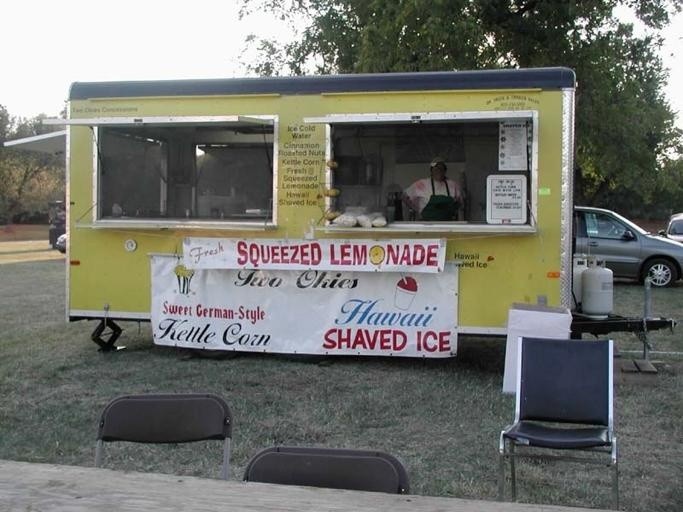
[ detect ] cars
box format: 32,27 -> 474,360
46,200 -> 66,252
574,202 -> 683,287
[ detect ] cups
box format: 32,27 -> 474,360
394,284 -> 417,309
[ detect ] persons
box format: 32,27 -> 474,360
401,157 -> 466,221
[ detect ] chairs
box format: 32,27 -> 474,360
496,335 -> 622,509
93,394 -> 234,481
243,446 -> 412,496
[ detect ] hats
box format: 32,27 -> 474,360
430,157 -> 447,168
